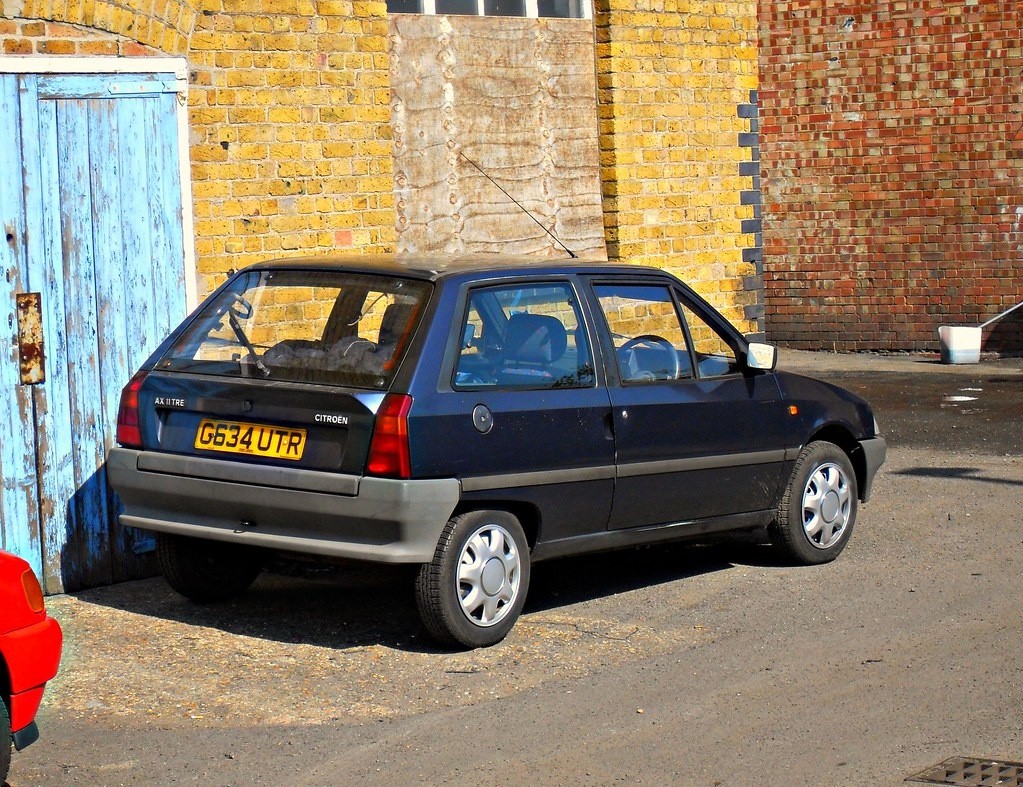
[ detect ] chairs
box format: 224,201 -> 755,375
265,302 -> 570,389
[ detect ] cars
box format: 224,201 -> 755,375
105,149 -> 886,650
0,547 -> 64,787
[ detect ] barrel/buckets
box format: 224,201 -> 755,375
938,326 -> 982,365
